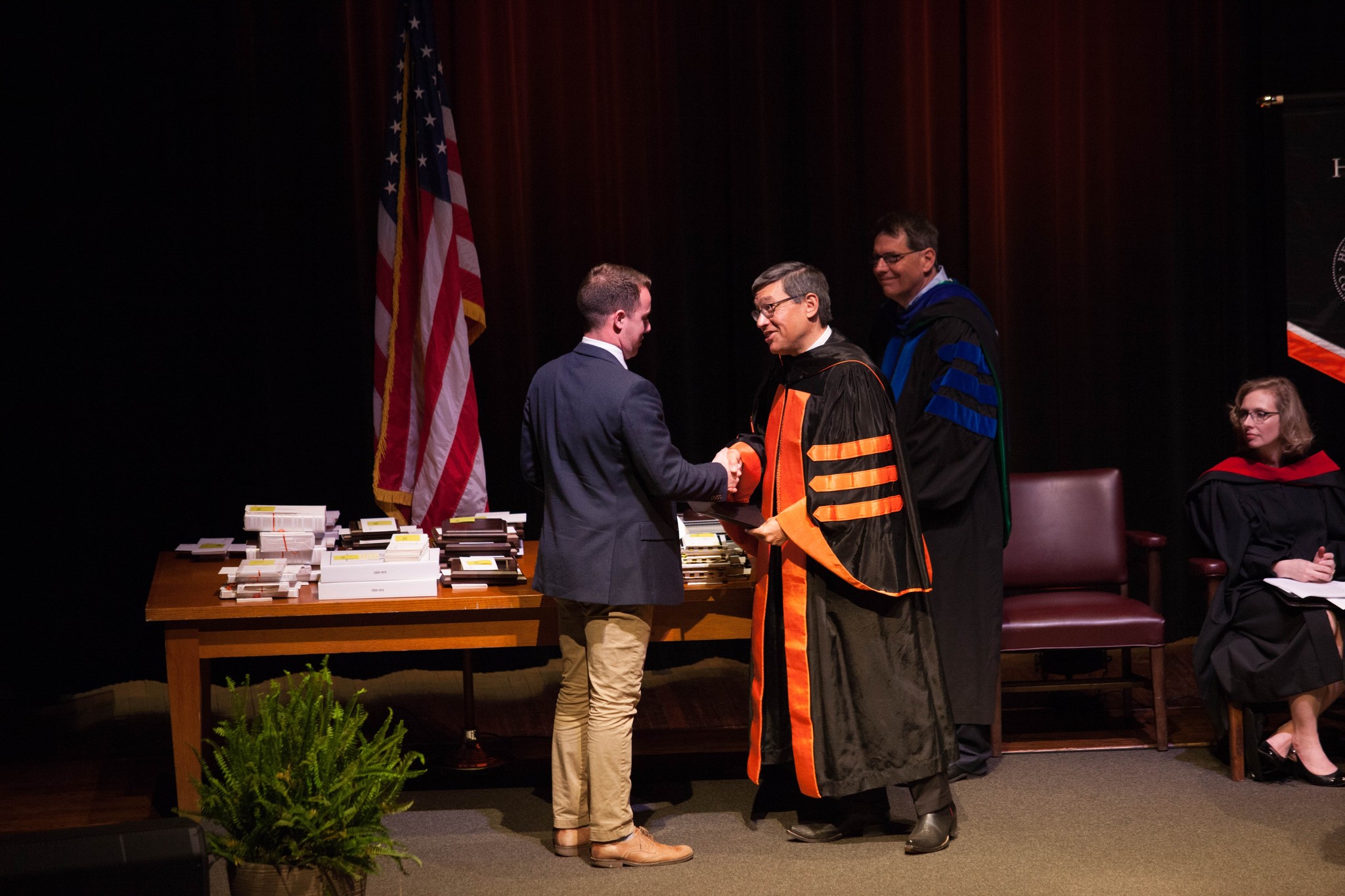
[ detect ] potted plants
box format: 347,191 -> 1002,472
169,641 -> 428,896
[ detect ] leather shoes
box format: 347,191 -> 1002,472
589,826 -> 693,867
554,825 -> 592,856
786,808 -> 890,842
904,799 -> 958,852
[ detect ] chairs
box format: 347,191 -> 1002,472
1188,548 -> 1345,784
986,456 -> 1175,757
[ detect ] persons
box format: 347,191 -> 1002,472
724,261 -> 957,855
1180,377 -> 1345,787
515,263 -> 743,870
869,217 -> 1017,785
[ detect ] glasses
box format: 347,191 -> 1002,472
1237,408 -> 1280,424
751,293 -> 807,322
870,248 -> 927,264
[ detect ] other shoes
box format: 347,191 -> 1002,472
947,764 -> 987,784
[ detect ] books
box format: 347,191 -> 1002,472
192,504 -> 528,604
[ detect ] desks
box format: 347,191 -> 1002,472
138,535 -> 757,822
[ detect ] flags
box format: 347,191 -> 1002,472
1282,99 -> 1345,384
368,0 -> 487,546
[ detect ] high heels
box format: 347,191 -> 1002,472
1287,749 -> 1345,787
1254,746 -> 1304,781
